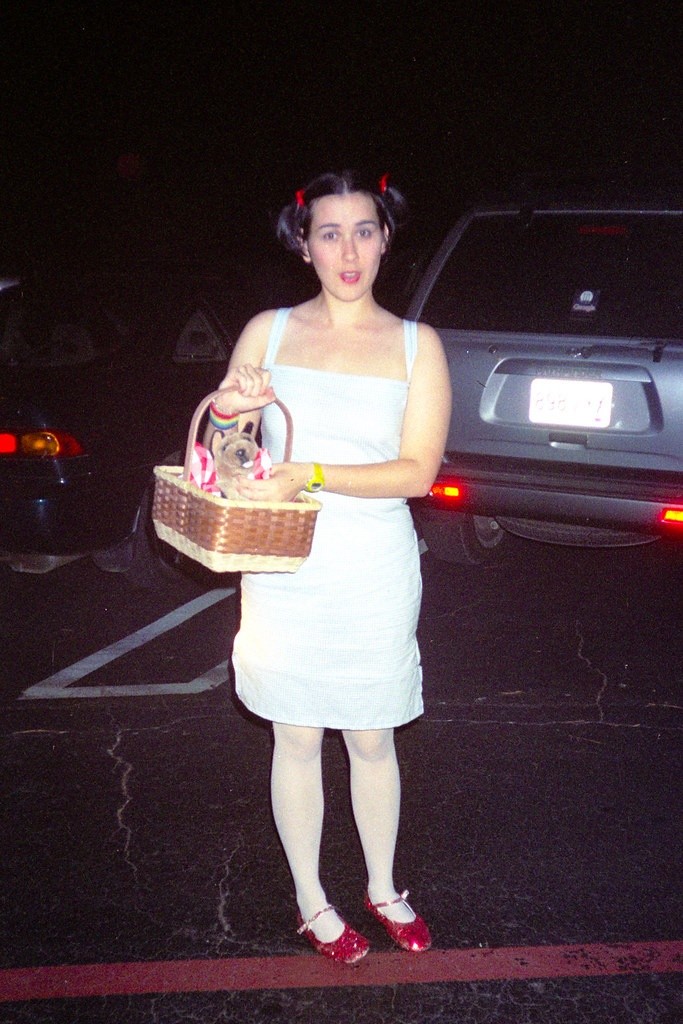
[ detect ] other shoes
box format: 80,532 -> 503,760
292,908 -> 370,964
362,892 -> 438,952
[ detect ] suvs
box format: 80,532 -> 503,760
398,195 -> 683,567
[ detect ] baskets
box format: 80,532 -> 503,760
152,383 -> 322,575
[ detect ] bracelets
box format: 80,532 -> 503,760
209,401 -> 239,431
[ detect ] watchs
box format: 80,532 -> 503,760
305,461 -> 325,493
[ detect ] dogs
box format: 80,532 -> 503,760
209,420 -> 260,502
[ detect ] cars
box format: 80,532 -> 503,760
0,263 -> 294,586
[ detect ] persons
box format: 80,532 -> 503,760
201,172 -> 453,963
